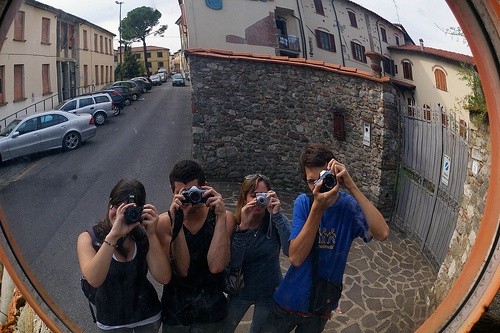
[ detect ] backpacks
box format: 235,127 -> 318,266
80,227 -> 100,305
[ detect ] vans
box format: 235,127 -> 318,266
158,68 -> 169,80
50,93 -> 116,126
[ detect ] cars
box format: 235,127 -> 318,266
79,77 -> 154,112
0,110 -> 98,166
156,73 -> 168,83
149,75 -> 163,86
171,73 -> 186,87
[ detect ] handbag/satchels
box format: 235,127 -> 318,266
311,278 -> 341,316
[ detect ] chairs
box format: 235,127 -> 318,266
25,120 -> 34,129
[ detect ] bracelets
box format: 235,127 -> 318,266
104,241 -> 114,247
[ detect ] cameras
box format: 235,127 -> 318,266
313,169 -> 337,193
181,186 -> 214,203
125,206 -> 147,223
255,192 -> 271,206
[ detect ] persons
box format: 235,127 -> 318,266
223,173 -> 291,333
257,143 -> 390,333
156,160 -> 235,333
77,178 -> 172,333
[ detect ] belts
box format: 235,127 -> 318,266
278,305 -> 334,319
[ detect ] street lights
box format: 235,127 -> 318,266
115,0 -> 124,81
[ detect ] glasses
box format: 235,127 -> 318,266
243,174 -> 270,181
